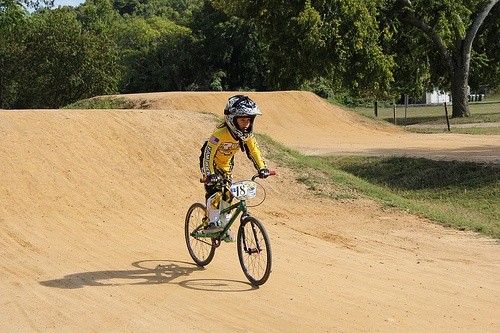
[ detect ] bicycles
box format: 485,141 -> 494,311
185,170 -> 275,285
